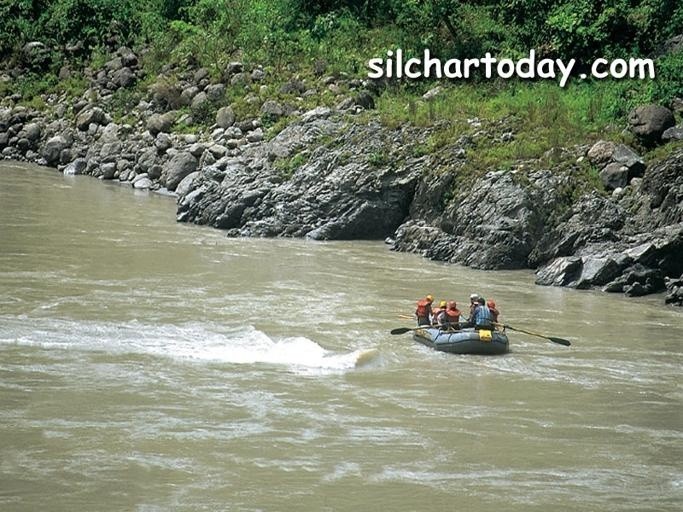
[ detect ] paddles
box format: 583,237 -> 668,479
391,322 -> 469,334
493,322 -> 571,347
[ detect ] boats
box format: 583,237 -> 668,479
412,324 -> 510,355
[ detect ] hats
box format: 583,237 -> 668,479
473,298 -> 484,305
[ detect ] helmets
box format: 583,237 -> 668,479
470,294 -> 478,303
450,301 -> 456,309
427,295 -> 434,302
488,301 -> 495,308
439,301 -> 446,308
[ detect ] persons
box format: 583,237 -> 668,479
432,301 -> 447,325
415,295 -> 433,326
487,298 -> 500,330
464,298 -> 492,330
446,300 -> 462,330
462,294 -> 482,329
